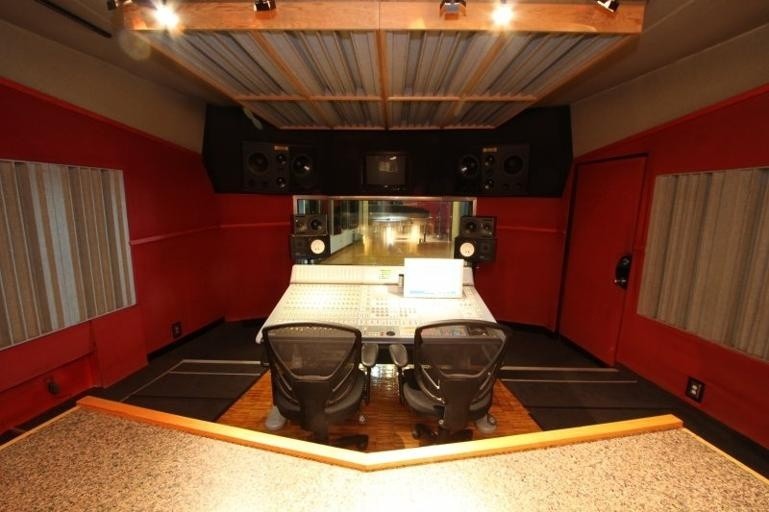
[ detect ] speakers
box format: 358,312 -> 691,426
461,217 -> 494,238
291,235 -> 330,258
242,141 -> 290,194
290,144 -> 320,193
454,235 -> 493,261
483,144 -> 529,195
294,214 -> 327,234
452,144 -> 483,195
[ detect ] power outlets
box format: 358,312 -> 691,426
686,380 -> 703,401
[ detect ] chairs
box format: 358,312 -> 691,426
263,322 -> 379,450
389,319 -> 513,442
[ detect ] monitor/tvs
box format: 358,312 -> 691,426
363,150 -> 408,191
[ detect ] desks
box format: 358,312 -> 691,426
256,264 -> 506,361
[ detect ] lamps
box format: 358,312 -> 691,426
597,0 -> 621,13
438,1 -> 468,16
252,0 -> 277,13
131,0 -> 165,10
